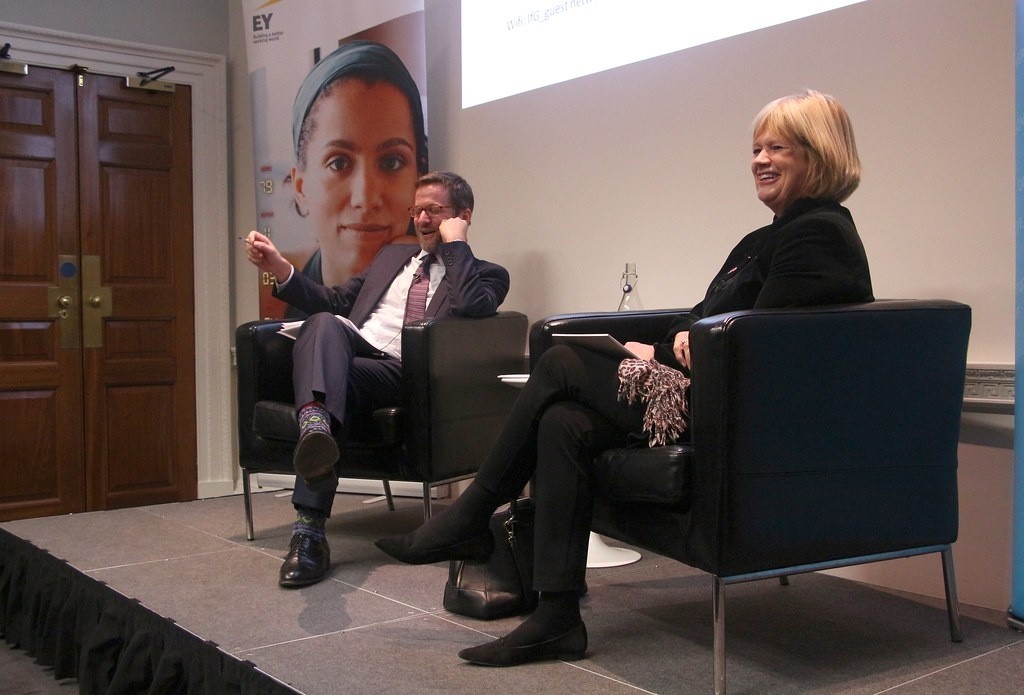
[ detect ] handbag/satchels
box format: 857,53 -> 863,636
442,499 -> 539,620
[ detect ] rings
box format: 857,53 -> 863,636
681,340 -> 687,346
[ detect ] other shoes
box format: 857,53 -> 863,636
458,620 -> 587,665
374,527 -> 496,565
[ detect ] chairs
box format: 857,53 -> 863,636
529,298 -> 973,695
235,310 -> 529,543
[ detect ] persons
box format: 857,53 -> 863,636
372,91 -> 872,666
283,41 -> 427,321
245,171 -> 509,587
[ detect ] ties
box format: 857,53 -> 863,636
403,253 -> 437,325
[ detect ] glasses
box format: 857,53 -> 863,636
409,205 -> 456,218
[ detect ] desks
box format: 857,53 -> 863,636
500,376 -> 641,567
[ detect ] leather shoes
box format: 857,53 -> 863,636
293,429 -> 339,492
279,533 -> 331,586
